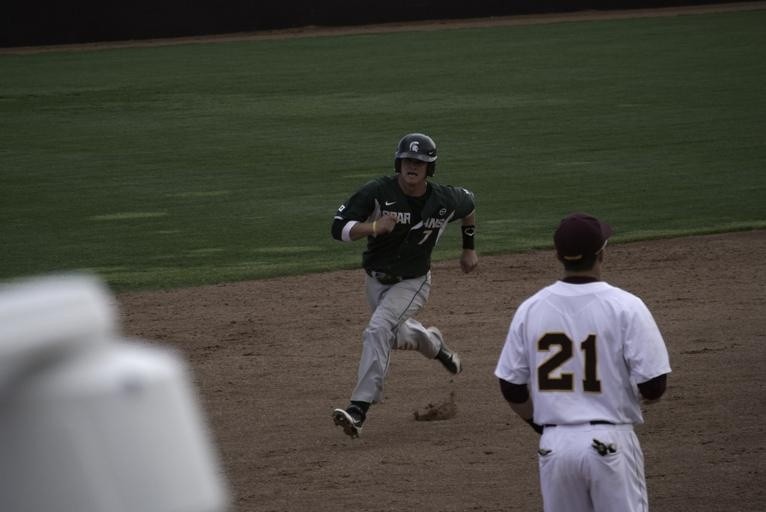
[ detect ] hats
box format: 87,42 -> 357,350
553,211 -> 611,260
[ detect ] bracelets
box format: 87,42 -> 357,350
524,417 -> 545,435
459,222 -> 477,251
367,219 -> 379,237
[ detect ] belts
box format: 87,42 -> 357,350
546,419 -> 613,429
364,266 -> 419,283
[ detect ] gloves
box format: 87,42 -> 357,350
527,415 -> 543,437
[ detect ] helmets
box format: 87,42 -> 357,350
393,133 -> 440,179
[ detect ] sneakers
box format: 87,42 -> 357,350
427,326 -> 461,373
326,402 -> 367,441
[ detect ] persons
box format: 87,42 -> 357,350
327,132 -> 481,438
493,212 -> 675,511
